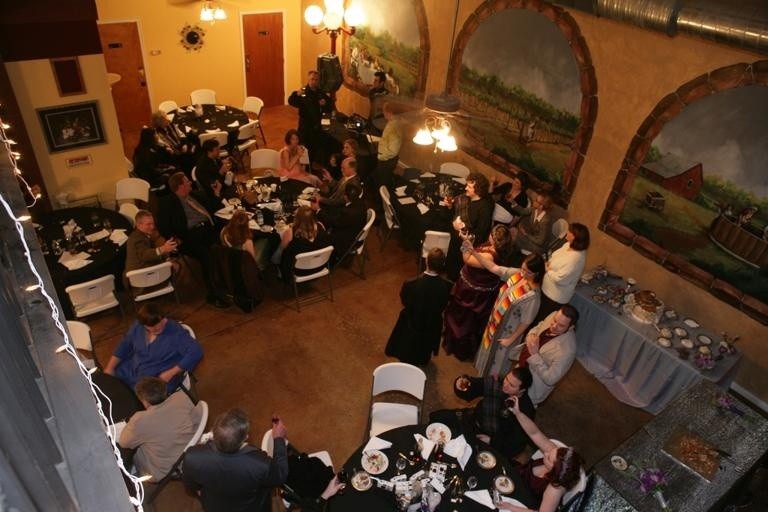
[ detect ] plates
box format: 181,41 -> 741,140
656,327 -> 712,349
259,225 -> 273,232
592,282 -> 622,308
349,422 -> 515,512
609,455 -> 627,470
302,186 -> 320,194
663,310 -> 679,321
214,196 -> 253,222
682,316 -> 702,328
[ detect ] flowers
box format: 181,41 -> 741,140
620,452 -> 671,509
712,390 -> 758,424
693,350 -> 724,370
717,334 -> 742,354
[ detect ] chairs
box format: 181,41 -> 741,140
531,438 -> 586,510
63,87 -> 569,328
368,362 -> 425,453
262,430 -> 336,508
65,320 -> 209,503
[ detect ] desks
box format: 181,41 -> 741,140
326,424 -> 530,512
554,377 -> 767,511
566,269 -> 744,417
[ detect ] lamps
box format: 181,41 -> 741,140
303,0 -> 365,109
411,1 -> 460,153
201,0 -> 226,21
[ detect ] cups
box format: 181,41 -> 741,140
244,179 -> 280,203
468,234 -> 475,243
460,375 -> 469,389
91,215 -> 111,229
271,413 -> 280,423
418,179 -> 461,210
500,400 -> 513,419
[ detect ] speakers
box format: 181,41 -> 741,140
317,51 -> 344,94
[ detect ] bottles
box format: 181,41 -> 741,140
337,467 -> 347,494
257,211 -> 264,225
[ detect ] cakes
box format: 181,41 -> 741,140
624,289 -> 665,322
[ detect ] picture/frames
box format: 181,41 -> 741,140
48,56 -> 87,98
33,98 -> 108,155
